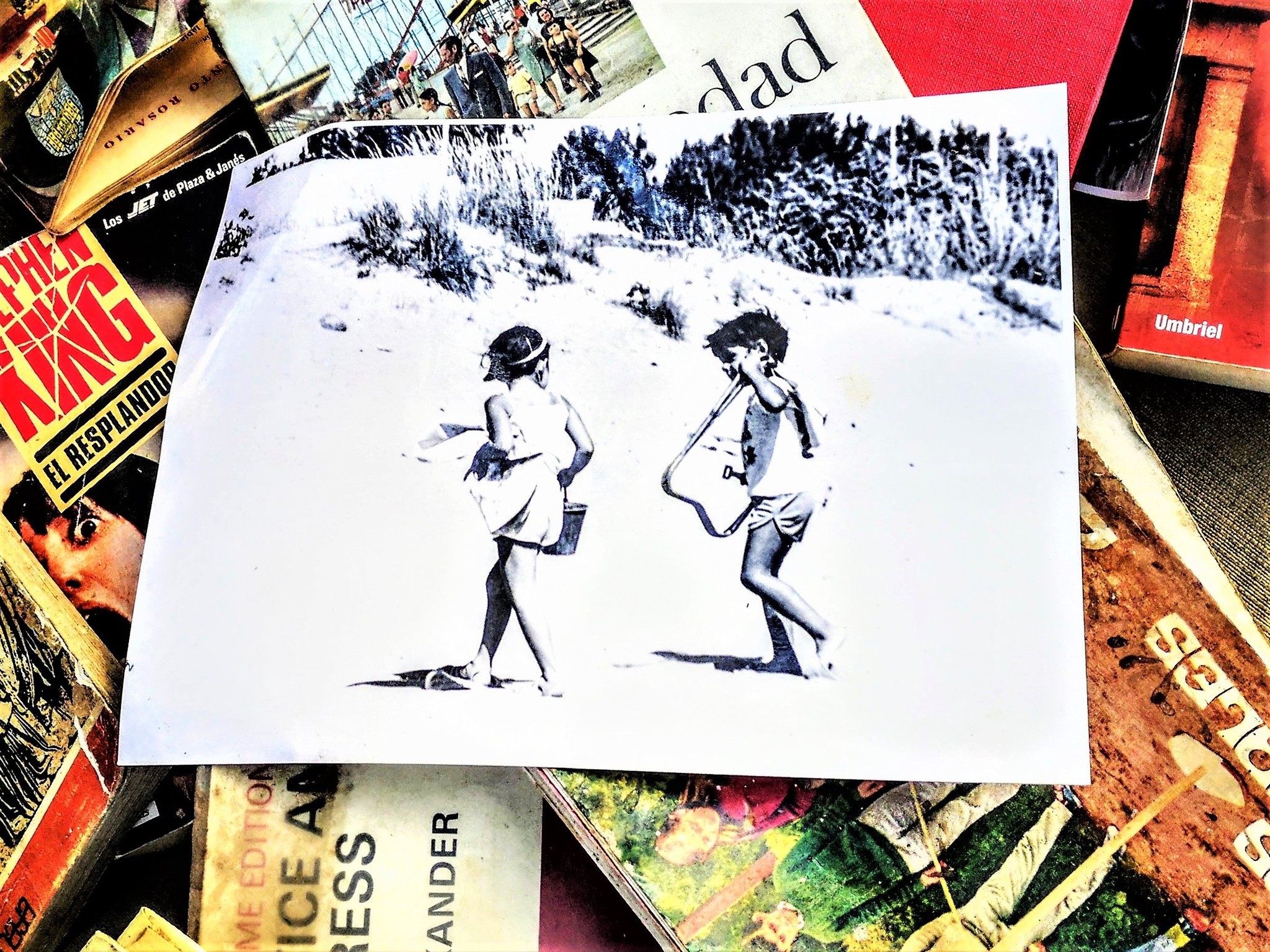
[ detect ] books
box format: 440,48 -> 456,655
528,429 -> 1270,952
0,0 -> 243,234
0,511 -> 200,952
1072,0 -> 1270,394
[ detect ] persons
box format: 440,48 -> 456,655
701,304 -> 843,679
347,0 -> 602,127
654,775 -> 1209,951
433,326 -> 595,698
5,453 -> 164,657
